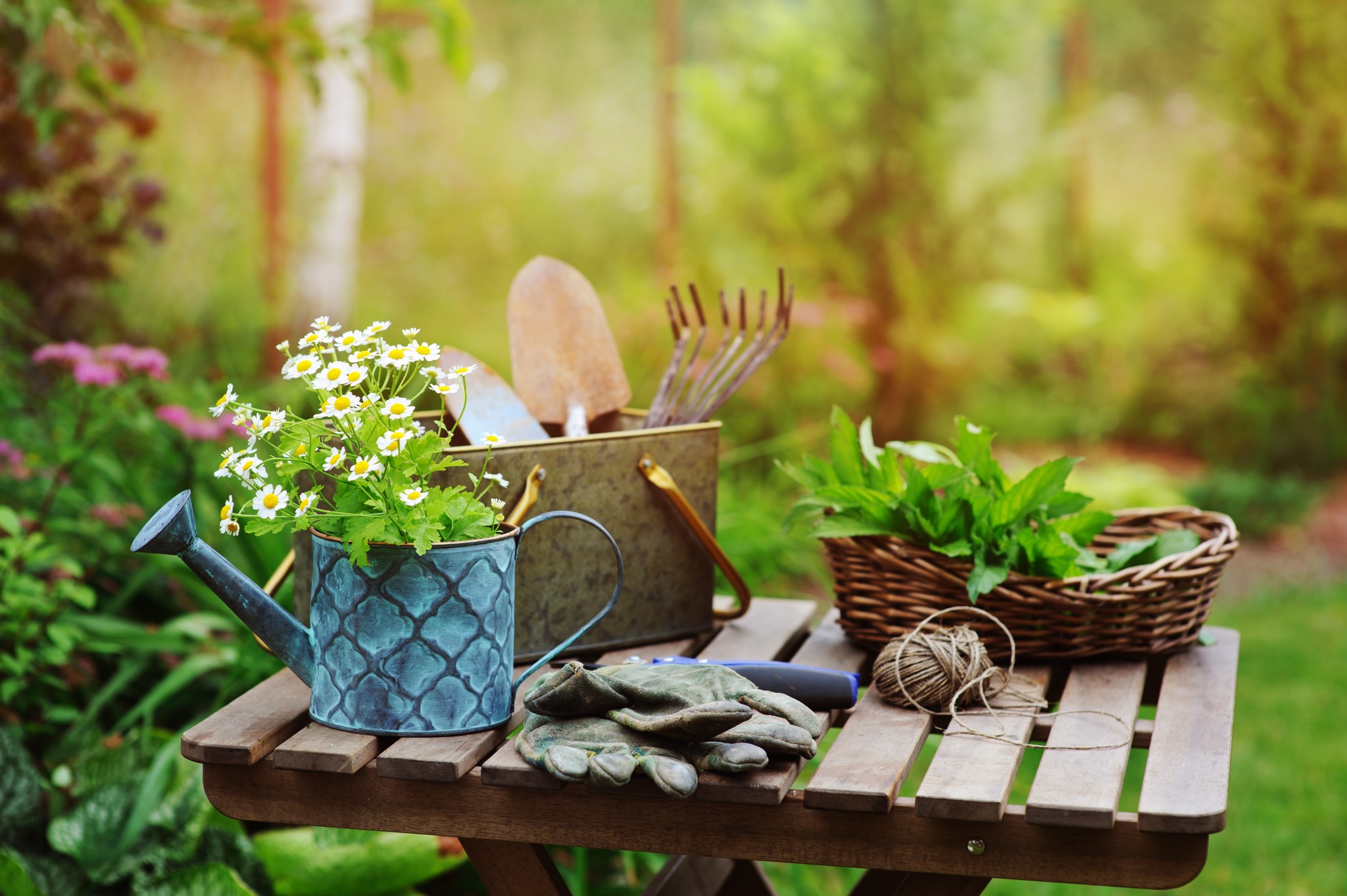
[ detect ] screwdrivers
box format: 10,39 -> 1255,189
550,657 -> 861,709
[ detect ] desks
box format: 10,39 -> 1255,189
180,594 -> 1239,896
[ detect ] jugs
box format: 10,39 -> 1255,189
128,488 -> 624,735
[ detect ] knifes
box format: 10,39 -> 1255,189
434,344 -> 549,445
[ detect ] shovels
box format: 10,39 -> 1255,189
438,349 -> 550,446
509,258 -> 630,438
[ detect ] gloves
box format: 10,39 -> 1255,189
513,659 -> 821,800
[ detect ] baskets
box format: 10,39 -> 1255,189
822,505 -> 1239,662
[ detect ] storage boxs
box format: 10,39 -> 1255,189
295,408 -> 724,664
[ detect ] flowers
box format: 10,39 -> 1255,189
208,317 -> 509,569
